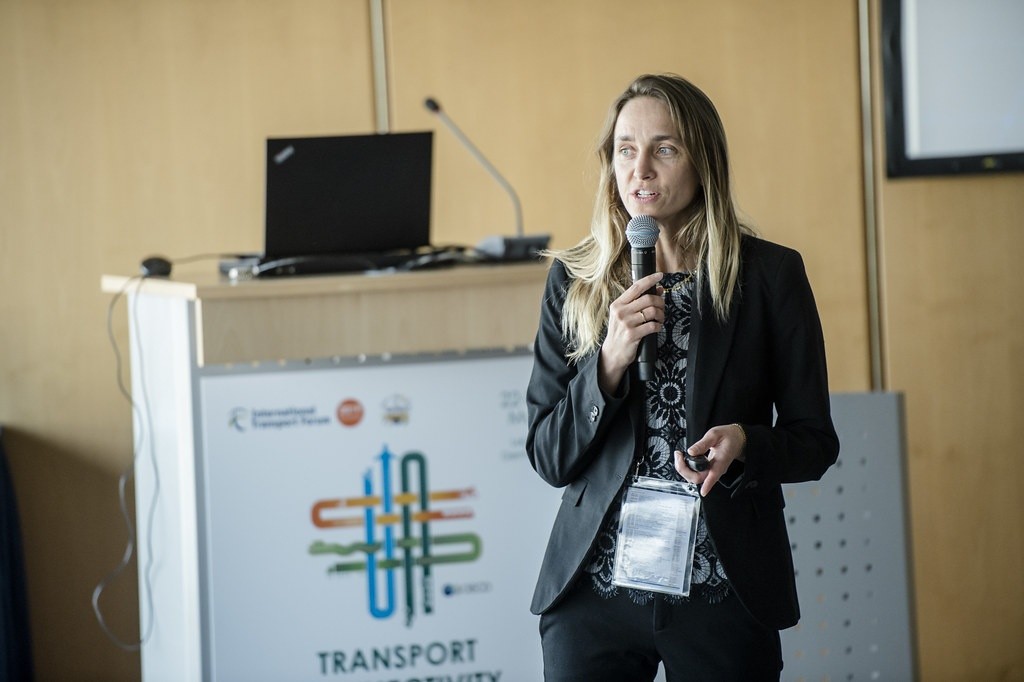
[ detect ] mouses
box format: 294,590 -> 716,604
139,258 -> 172,278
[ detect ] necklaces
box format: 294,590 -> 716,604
660,270 -> 696,294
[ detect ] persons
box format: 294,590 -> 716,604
523,72 -> 841,681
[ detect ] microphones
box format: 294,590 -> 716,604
625,214 -> 661,383
425,98 -> 552,263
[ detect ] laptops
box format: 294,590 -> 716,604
218,132 -> 436,279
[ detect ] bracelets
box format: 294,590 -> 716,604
731,423 -> 746,448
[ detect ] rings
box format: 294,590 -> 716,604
641,310 -> 646,323
